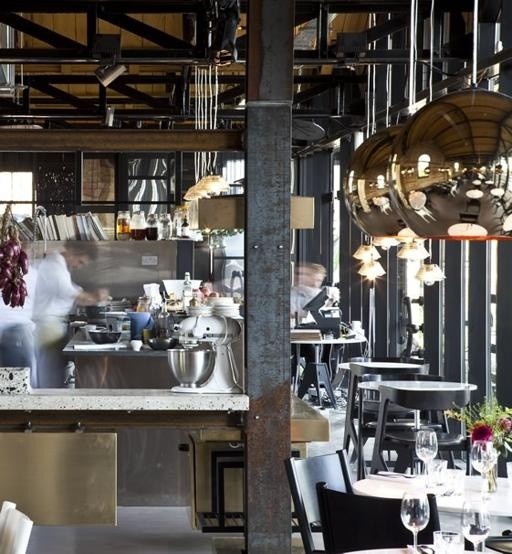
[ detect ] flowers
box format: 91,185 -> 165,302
445,392 -> 512,449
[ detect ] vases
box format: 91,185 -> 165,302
483,445 -> 501,491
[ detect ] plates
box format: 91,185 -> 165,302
187,303 -> 240,318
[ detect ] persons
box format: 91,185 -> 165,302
290,263 -> 337,402
30,233 -> 108,389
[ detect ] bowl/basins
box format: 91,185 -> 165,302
147,336 -> 177,350
88,329 -> 122,344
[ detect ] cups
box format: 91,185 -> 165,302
351,320 -> 361,334
143,329 -> 154,344
430,459 -> 466,497
85,325 -> 96,340
433,530 -> 462,554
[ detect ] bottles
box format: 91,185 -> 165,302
116,210 -> 190,241
158,271 -> 220,337
136,296 -> 153,313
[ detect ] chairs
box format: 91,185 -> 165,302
290,336 -> 478,470
285,449 -> 441,554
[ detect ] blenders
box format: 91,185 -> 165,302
167,314 -> 243,395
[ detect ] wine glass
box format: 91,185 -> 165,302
401,489 -> 431,554
461,500 -> 491,554
470,440 -> 496,503
415,430 -> 438,491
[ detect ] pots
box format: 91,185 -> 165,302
86,295 -> 127,319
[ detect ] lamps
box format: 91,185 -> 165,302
352,13 -> 447,286
94,63 -> 127,88
183,64 -> 231,200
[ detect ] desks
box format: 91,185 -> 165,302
352,471 -> 512,516
